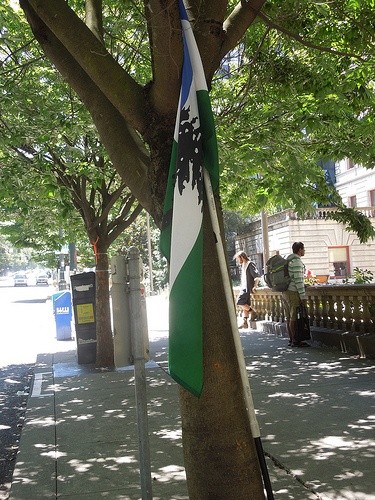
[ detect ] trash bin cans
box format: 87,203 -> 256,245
52,292 -> 75,341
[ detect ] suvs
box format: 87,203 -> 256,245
13,274 -> 29,287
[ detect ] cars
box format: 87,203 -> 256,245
36,274 -> 49,286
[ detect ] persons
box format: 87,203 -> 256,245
282,241 -> 311,348
233,251 -> 261,329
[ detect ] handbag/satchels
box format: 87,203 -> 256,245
293,305 -> 311,343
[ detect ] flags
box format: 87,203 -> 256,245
159,0 -> 219,399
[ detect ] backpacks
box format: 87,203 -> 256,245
263,254 -> 302,291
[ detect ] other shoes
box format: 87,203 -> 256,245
288,341 -> 311,347
250,310 -> 257,320
239,323 -> 248,329
